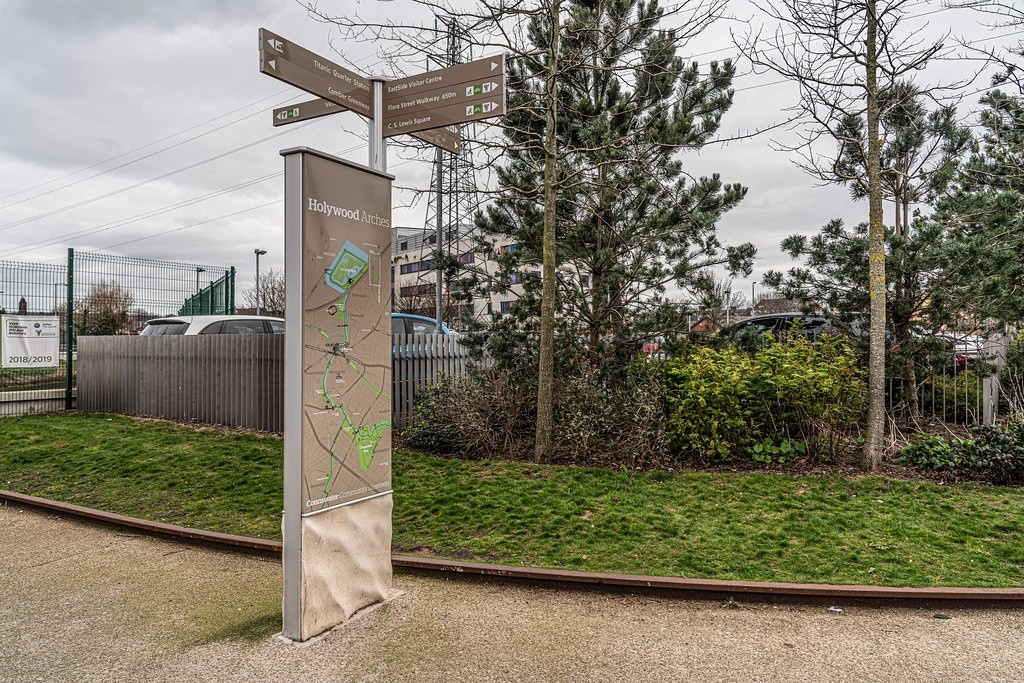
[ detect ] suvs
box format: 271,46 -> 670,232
136,315 -> 286,337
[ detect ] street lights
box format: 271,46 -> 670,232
254,248 -> 267,316
196,267 -> 205,294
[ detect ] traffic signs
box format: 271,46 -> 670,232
257,27 -> 507,156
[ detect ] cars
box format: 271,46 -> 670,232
686,310 -> 984,399
388,312 -> 458,356
